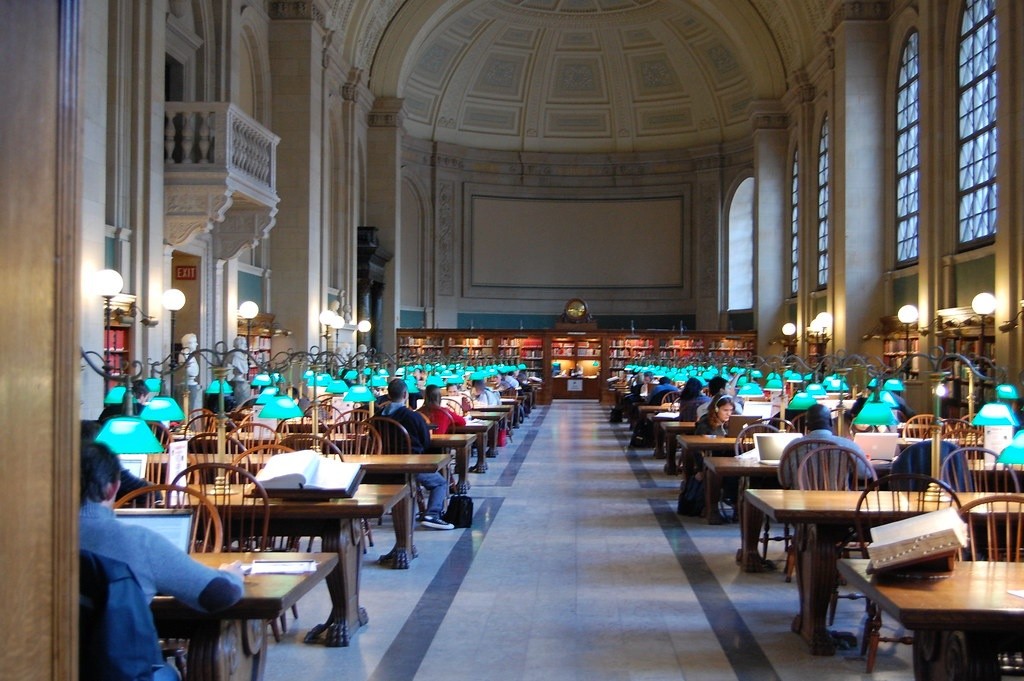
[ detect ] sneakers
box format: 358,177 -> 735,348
421,515 -> 455,529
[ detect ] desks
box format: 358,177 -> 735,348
148,454 -> 451,569
151,552 -> 339,681
745,488 -> 1024,657
835,558 -> 1024,681
608,383 -> 983,490
158,482 -> 410,647
702,455 -> 1024,573
172,429 -> 477,495
281,382 -> 539,474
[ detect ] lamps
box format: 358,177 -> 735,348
80,269 -> 527,454
593,360 -> 599,367
623,293 -> 1024,464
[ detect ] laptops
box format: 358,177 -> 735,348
853,432 -> 899,461
112,508 -> 197,602
726,416 -> 764,439
753,432 -> 803,465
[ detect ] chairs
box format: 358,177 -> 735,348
108,387 -> 518,681
661,384 -> 1024,673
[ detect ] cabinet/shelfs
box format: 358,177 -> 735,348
396,328 -> 758,405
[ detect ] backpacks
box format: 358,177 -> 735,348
632,418 -> 653,447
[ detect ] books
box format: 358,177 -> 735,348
578,341 -> 601,356
551,340 -> 573,357
865,506 -> 969,575
611,358 -> 631,368
244,451 -> 366,498
660,340 -> 704,357
709,340 -> 753,357
400,336 -> 542,367
881,338 -> 995,425
609,338 -> 654,358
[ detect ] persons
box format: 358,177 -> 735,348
339,368 -> 355,387
768,382 -> 919,490
508,365 -> 532,396
369,378 -> 454,529
488,370 -> 513,390
570,364 -> 583,377
284,386 -> 329,418
78,442 -> 245,681
98,380 -> 171,444
413,368 -> 423,380
471,378 -> 507,446
610,367 -> 748,518
446,383 -> 471,417
207,381 -> 238,413
416,384 -> 466,494
81,420 -> 163,508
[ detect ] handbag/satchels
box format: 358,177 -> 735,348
610,407 -> 623,422
441,495 -> 473,528
676,474 -> 706,516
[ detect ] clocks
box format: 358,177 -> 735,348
565,297 -> 589,321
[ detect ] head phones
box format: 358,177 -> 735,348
714,395 -> 734,412
